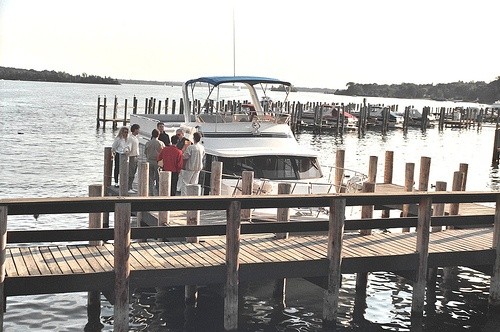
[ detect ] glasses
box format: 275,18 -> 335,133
176,134 -> 182,136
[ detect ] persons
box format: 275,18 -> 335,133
182,132 -> 205,196
126,124 -> 140,194
156,135 -> 184,196
112,126 -> 129,188
144,129 -> 166,196
151,122 -> 170,146
176,128 -> 193,192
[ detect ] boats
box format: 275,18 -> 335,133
408,108 -> 436,121
370,106 -> 404,124
323,104 -> 359,126
128,74 -> 371,222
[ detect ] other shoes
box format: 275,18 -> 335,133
127,189 -> 136,194
114,184 -> 119,188
131,188 -> 138,192
